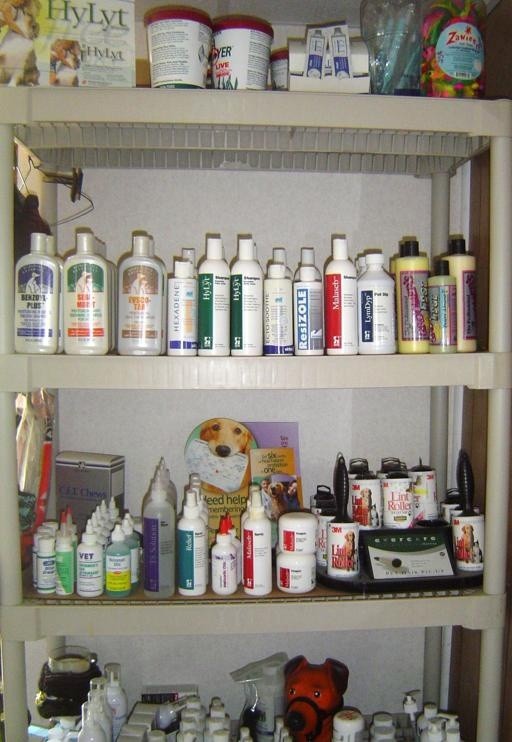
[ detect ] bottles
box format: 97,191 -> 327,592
404,692 -> 443,742
329,710 -> 396,742
11,226 -> 478,358
77,660 -> 293,742
46,715 -> 78,742
29,457 -> 273,601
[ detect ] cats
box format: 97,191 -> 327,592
472,539 -> 482,563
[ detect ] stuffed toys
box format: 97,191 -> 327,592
283,655 -> 349,742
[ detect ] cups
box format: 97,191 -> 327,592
270,49 -> 290,91
309,465 -> 485,578
143,3 -> 215,89
210,14 -> 275,90
49,645 -> 95,674
359,0 -> 422,95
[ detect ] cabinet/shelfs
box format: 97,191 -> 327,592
1,84 -> 511,742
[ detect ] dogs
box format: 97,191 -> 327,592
199,418 -> 255,495
458,524 -> 474,552
267,482 -> 288,521
